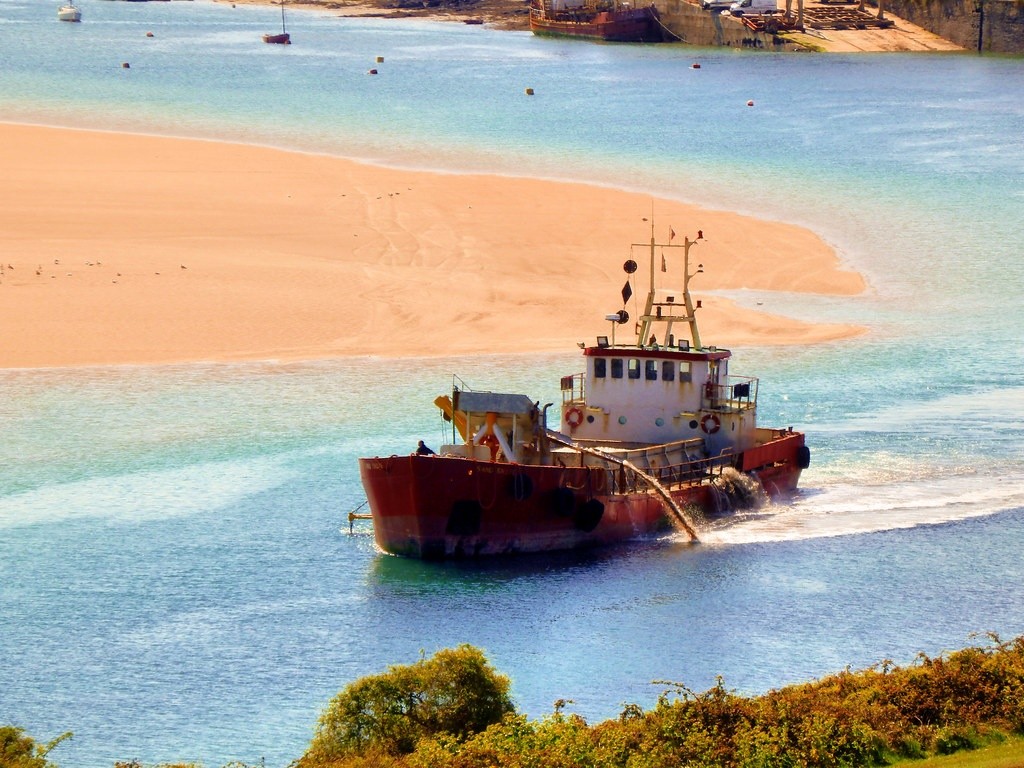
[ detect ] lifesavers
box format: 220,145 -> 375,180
797,446 -> 811,469
508,474 -> 606,534
706,381 -> 713,399
701,415 -> 720,434
479,434 -> 500,454
564,408 -> 583,427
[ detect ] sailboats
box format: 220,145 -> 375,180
262,1 -> 290,43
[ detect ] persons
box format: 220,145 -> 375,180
650,334 -> 656,347
415,441 -> 435,455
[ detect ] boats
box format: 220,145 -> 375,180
358,207 -> 811,563
58,0 -> 81,22
527,0 -> 664,43
463,19 -> 484,24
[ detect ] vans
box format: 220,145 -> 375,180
702,0 -> 778,18
